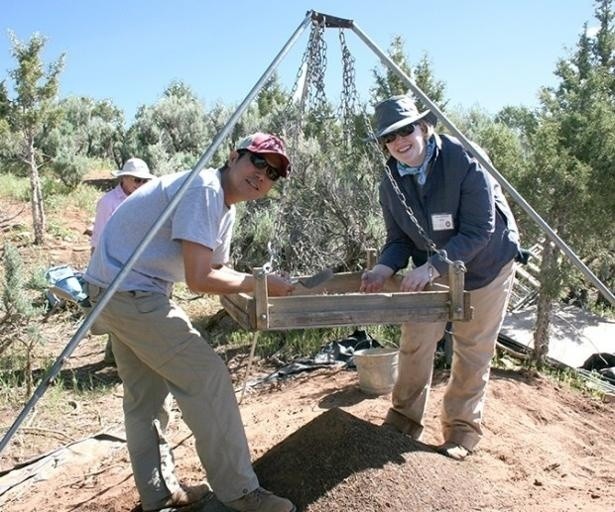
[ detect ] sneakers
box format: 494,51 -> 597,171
143,484 -> 209,512
438,440 -> 468,460
224,485 -> 296,512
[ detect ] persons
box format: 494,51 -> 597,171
358,98 -> 520,459
86,130 -> 297,512
90,158 -> 156,365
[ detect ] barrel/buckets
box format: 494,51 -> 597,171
352,337 -> 400,394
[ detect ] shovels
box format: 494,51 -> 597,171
291,268 -> 333,289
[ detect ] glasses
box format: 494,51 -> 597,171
382,123 -> 417,142
250,151 -> 280,181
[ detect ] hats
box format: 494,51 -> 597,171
236,134 -> 290,164
112,158 -> 157,180
362,96 -> 431,142
